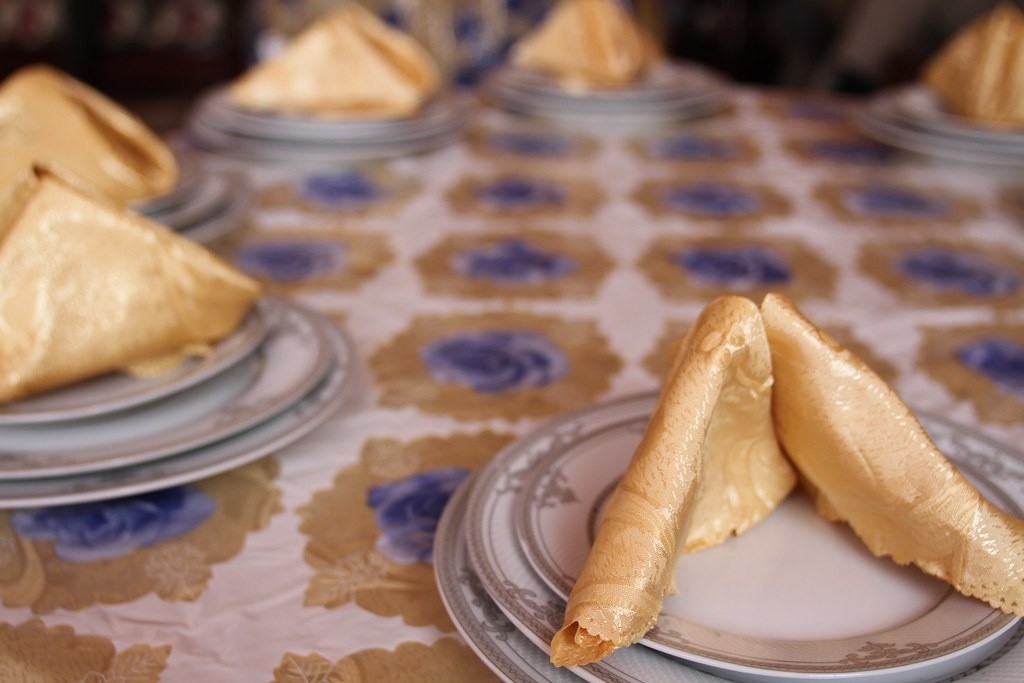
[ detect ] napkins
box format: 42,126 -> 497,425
506,1 -> 663,91
0,164 -> 270,416
0,61 -> 180,246
230,4 -> 439,122
928,7 -> 1023,135
547,284 -> 1024,669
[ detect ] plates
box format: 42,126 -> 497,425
851,83 -> 1023,169
430,392 -> 1024,683
190,65 -> 726,164
2,151 -> 348,509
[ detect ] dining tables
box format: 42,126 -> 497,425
0,54 -> 1024,683
